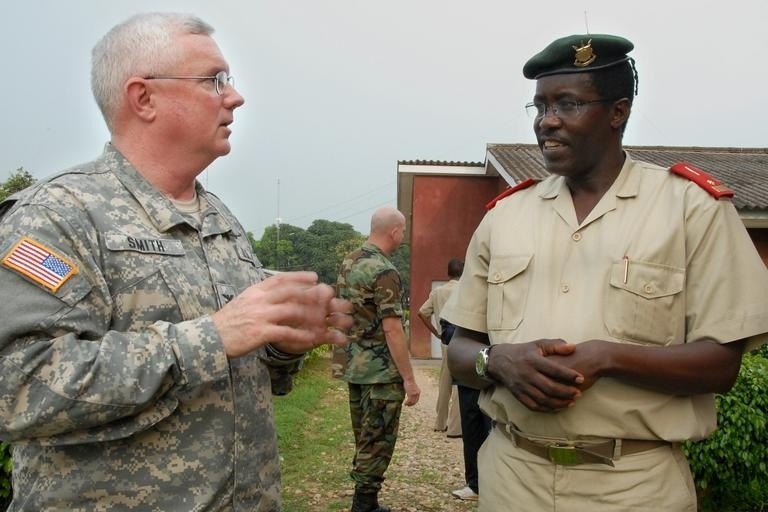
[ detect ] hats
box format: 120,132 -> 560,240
522,34 -> 634,79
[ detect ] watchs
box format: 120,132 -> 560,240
476,344 -> 491,377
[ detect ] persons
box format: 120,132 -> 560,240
439,33 -> 768,511
331,207 -> 421,512
0,12 -> 354,512
417,257 -> 465,438
440,319 -> 492,502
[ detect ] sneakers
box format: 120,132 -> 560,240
451,486 -> 478,501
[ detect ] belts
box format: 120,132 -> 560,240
497,422 -> 670,465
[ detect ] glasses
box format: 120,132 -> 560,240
144,71 -> 234,95
526,97 -> 606,119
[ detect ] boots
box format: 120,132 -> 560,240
351,485 -> 392,511
